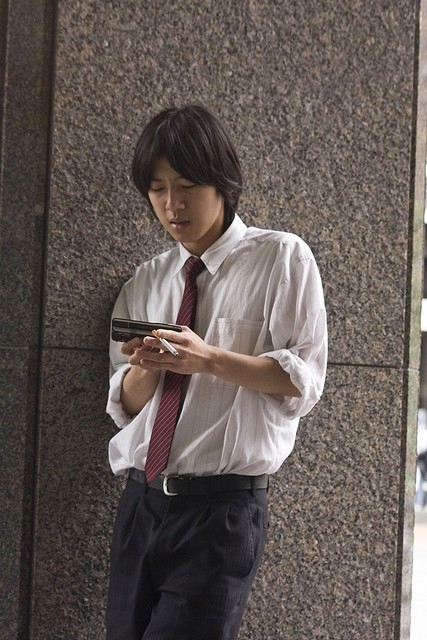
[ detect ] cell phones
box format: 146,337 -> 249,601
112,317 -> 183,343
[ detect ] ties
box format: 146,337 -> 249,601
145,257 -> 206,484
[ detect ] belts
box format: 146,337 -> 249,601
129,469 -> 268,497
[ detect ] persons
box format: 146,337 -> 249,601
103,105 -> 327,640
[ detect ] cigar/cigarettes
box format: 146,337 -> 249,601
152,330 -> 178,357
